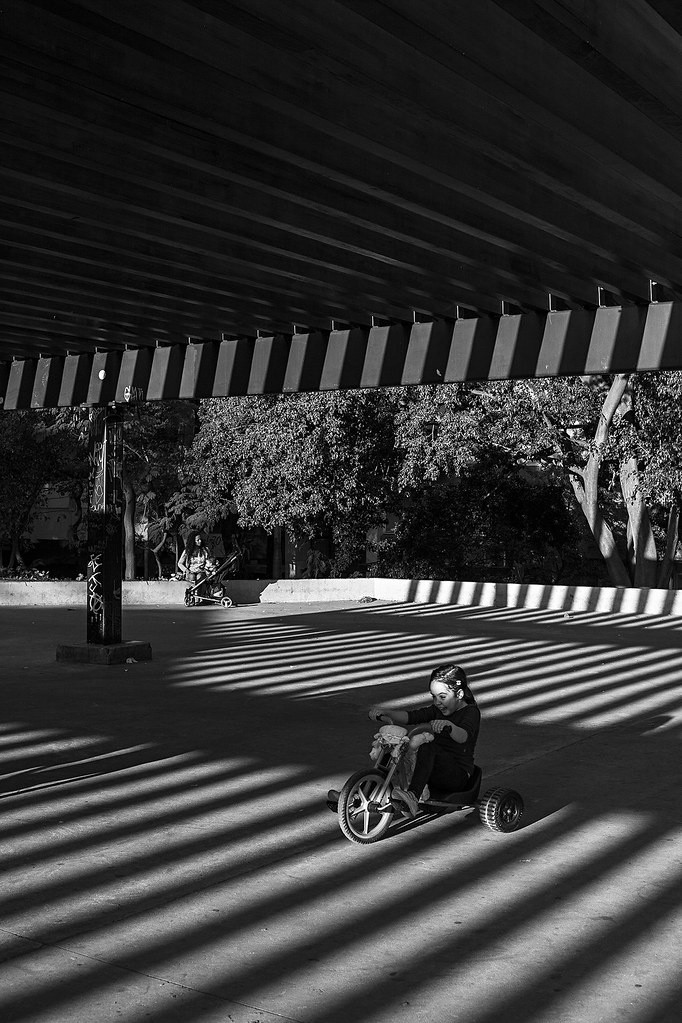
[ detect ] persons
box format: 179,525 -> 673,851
328,663 -> 481,819
178,531 -> 220,585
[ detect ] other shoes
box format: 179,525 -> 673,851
391,787 -> 418,819
328,790 -> 342,804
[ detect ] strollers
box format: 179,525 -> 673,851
183,550 -> 240,610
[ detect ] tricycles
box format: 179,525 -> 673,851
327,709 -> 524,846
